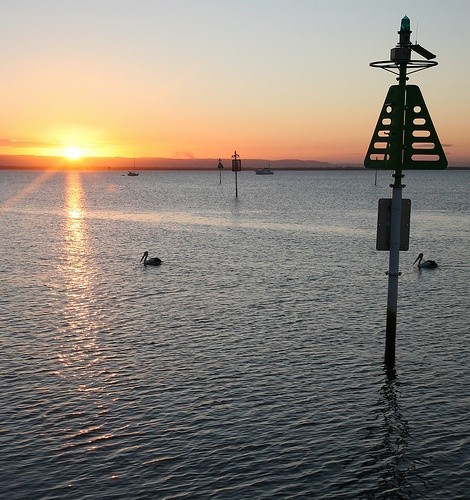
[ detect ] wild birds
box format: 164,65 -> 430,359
140,251 -> 162,266
413,253 -> 439,269
